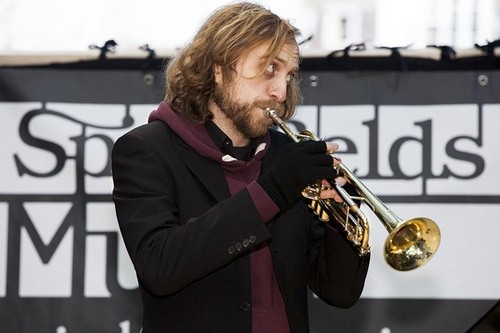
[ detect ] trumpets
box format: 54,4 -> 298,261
264,105 -> 441,272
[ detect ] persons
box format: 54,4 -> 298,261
109,1 -> 376,333
